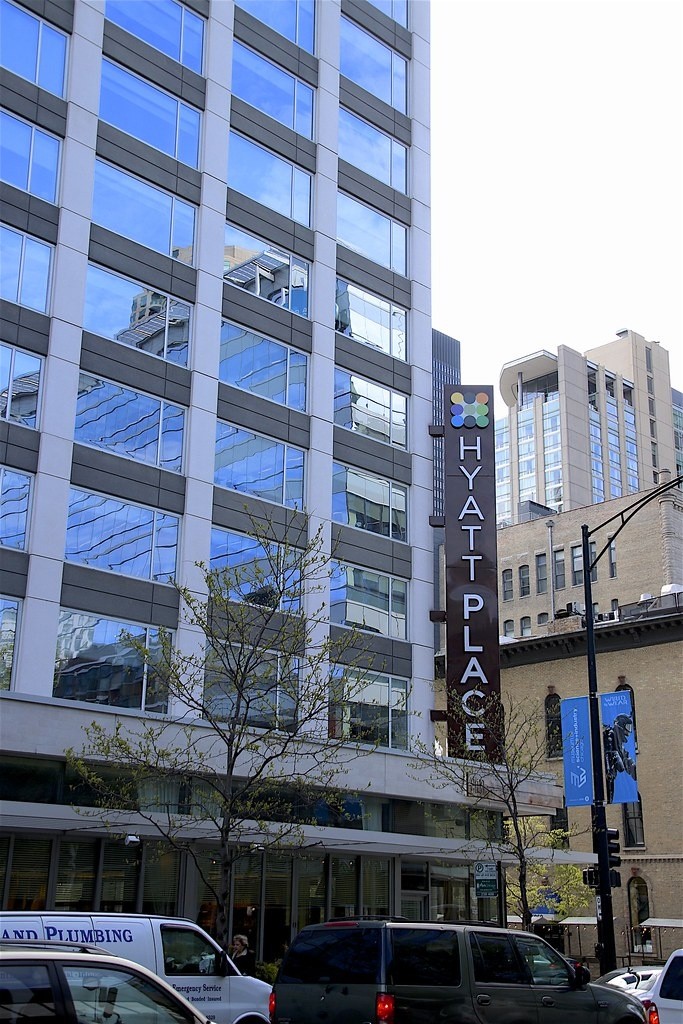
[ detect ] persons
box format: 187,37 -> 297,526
603,713 -> 636,804
581,957 -> 589,969
229,934 -> 256,976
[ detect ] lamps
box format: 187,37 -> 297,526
250,844 -> 264,855
125,834 -> 140,847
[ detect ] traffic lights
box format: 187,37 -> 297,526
605,826 -> 622,869
611,870 -> 622,888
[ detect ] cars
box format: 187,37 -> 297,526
527,951 -> 591,982
594,965 -> 665,993
640,951 -> 683,1024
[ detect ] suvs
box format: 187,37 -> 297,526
266,914 -> 649,1024
1,938 -> 217,1024
0,912 -> 276,1024
